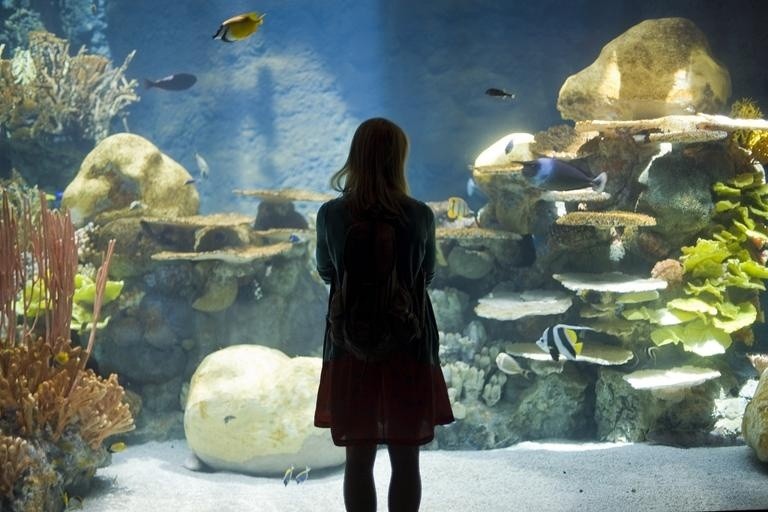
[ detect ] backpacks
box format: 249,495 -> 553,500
326,195 -> 427,366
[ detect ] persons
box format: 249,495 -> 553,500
317,114 -> 457,511
